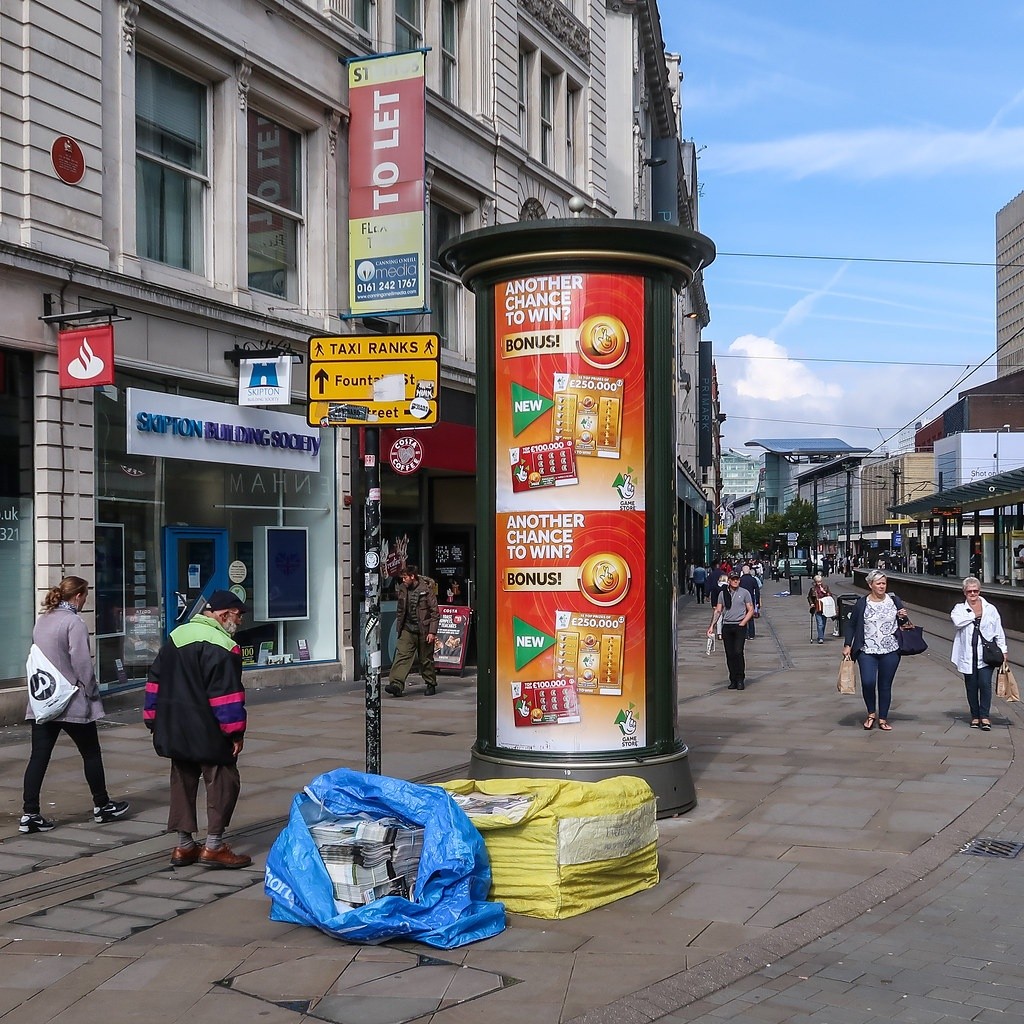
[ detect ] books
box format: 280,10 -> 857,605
308,813 -> 425,915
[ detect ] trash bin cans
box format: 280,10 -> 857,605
771,567 -> 777,580
837,595 -> 861,637
789,574 -> 802,595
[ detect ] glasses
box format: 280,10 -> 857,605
729,572 -> 739,575
228,611 -> 243,619
965,590 -> 979,593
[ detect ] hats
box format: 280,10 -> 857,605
727,570 -> 740,580
203,590 -> 247,614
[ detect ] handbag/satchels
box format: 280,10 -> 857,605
996,662 -> 1020,702
892,613 -> 928,655
26,644 -> 79,725
837,655 -> 855,694
983,638 -> 1004,667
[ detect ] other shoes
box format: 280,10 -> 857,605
728,683 -> 736,689
737,681 -> 744,690
980,720 -> 991,730
971,719 -> 980,728
817,638 -> 824,643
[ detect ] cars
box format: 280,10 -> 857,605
777,558 -> 824,579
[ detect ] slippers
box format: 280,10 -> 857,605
863,715 -> 877,729
878,721 -> 891,730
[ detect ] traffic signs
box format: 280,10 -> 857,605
309,360 -> 440,428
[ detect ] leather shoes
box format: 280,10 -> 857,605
171,842 -> 203,867
199,842 -> 252,868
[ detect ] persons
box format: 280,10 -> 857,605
680,541 -> 1024,605
15,576 -> 130,834
142,589 -> 252,871
384,567 -> 442,698
844,572 -> 907,732
951,576 -> 1007,732
808,573 -> 834,647
707,570 -> 755,691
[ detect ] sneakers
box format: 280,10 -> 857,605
425,684 -> 435,696
94,800 -> 130,823
385,685 -> 403,697
18,814 -> 55,833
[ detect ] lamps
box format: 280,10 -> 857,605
682,312 -> 698,325
640,157 -> 667,177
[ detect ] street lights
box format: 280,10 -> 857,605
997,424 -> 1011,474
